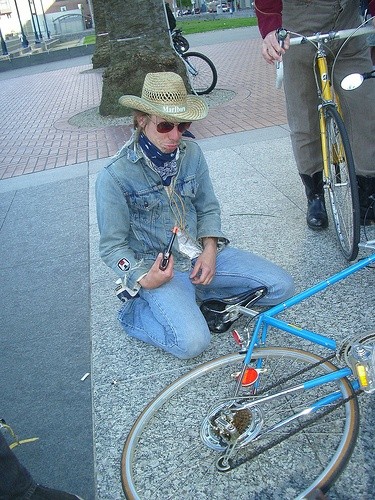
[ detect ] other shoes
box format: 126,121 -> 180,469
115,284 -> 140,303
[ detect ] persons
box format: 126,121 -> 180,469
253,0 -> 375,231
94,71 -> 295,359
0,429 -> 85,500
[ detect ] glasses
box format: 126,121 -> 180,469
146,113 -> 193,133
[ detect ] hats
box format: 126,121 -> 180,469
119,72 -> 210,123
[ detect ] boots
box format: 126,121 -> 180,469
357,175 -> 375,226
298,169 -> 329,231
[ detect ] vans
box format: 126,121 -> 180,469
216,5 -> 228,13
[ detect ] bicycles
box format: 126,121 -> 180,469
119,238 -> 375,499
275,16 -> 375,262
171,27 -> 217,96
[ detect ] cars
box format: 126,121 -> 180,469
183,10 -> 191,15
194,8 -> 200,14
